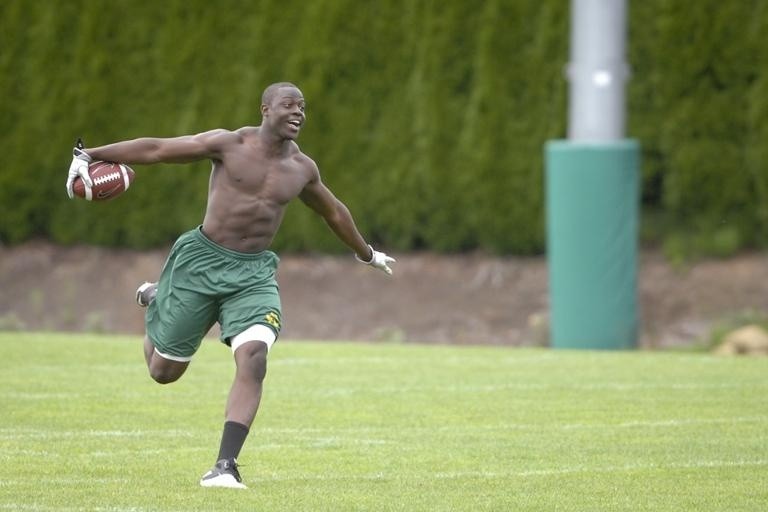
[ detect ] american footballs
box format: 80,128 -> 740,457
72,160 -> 136,200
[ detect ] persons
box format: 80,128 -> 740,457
66,82 -> 396,490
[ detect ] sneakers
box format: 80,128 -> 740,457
199,457 -> 248,489
135,281 -> 159,308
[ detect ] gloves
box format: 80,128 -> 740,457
354,244 -> 396,276
66,146 -> 94,201
